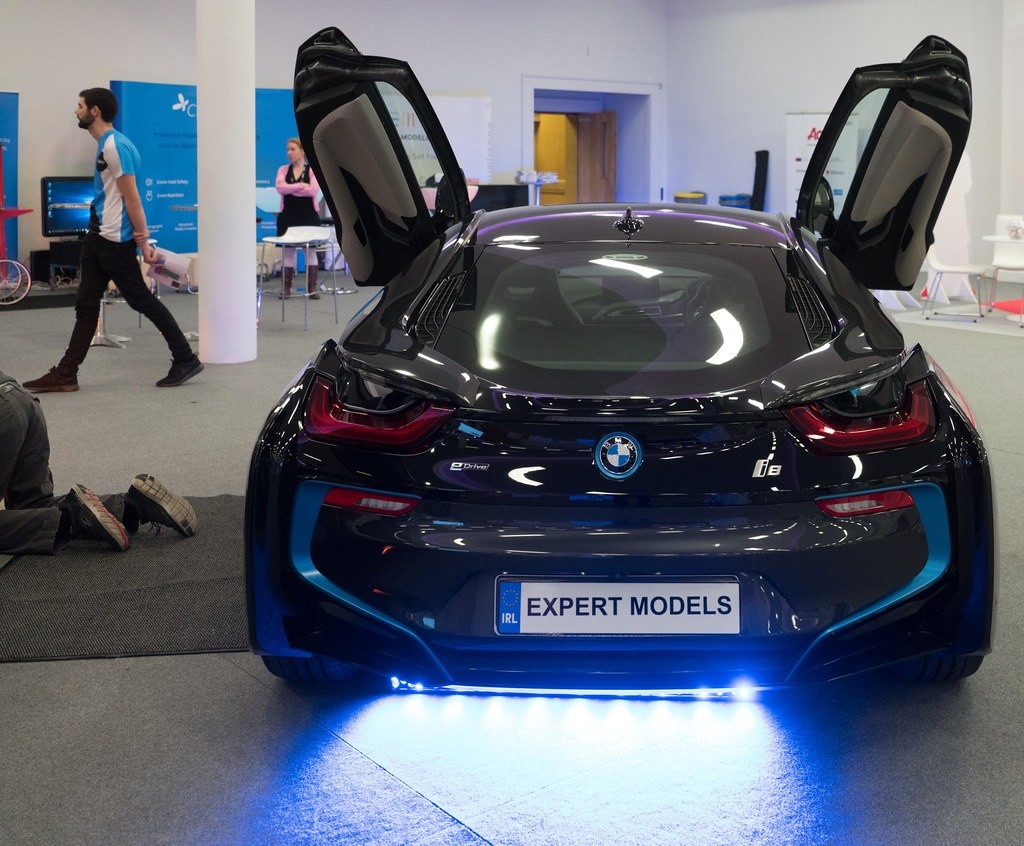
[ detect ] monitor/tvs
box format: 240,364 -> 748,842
41,176 -> 94,237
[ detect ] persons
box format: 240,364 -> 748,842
22,88 -> 204,392
0,369 -> 197,556
276,137 -> 320,299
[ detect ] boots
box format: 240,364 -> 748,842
278,266 -> 294,300
307,265 -> 320,299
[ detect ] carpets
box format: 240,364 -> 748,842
0,491 -> 255,663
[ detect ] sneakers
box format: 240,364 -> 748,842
132,473 -> 199,537
155,351 -> 204,388
22,366 -> 80,392
60,482 -> 130,552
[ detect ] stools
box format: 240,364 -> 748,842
256,226 -> 338,331
64,280 -> 131,350
318,249 -> 358,294
134,239 -> 160,327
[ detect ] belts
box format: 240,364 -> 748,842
0,380 -> 21,396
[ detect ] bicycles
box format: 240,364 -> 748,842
0,259 -> 31,305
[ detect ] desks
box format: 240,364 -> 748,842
981,235 -> 1024,244
168,204 -> 197,295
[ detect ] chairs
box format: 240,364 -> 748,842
922,243 -> 988,322
490,248 -> 582,327
987,214 -> 1024,328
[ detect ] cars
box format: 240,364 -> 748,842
243,27 -> 998,700
